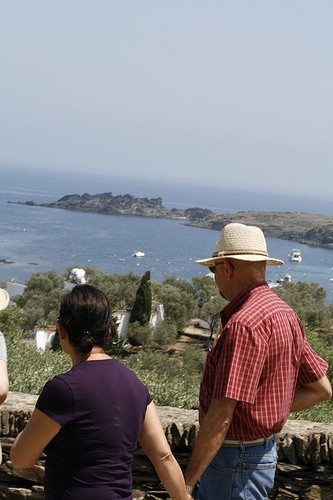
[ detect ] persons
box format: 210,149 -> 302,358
182,223 -> 332,500
0,289 -> 9,404
10,284 -> 197,500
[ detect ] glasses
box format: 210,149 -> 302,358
208,260 -> 235,272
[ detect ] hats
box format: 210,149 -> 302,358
194,222 -> 284,266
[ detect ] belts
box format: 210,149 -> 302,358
223,433 -> 274,449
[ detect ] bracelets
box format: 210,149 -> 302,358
185,485 -> 193,492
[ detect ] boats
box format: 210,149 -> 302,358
288,249 -> 303,263
